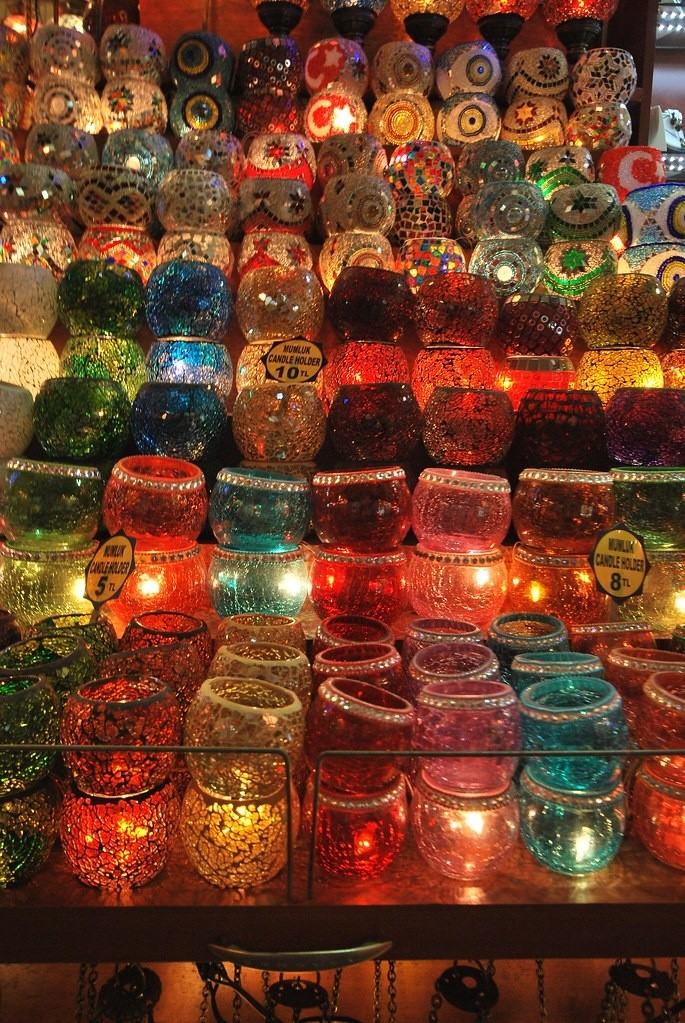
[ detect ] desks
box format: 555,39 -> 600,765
1,842 -> 685,1023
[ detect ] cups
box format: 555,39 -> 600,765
0,0 -> 684,879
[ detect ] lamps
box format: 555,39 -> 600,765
0,0 -> 684,893
